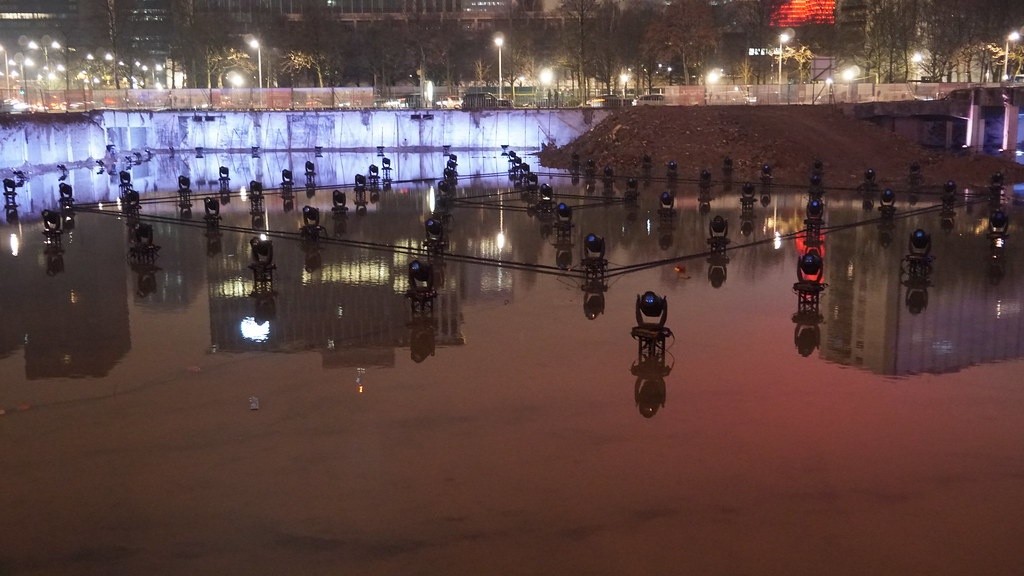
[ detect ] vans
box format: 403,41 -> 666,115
632,95 -> 666,106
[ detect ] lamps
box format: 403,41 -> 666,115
792,160 -> 825,293
280,146 -> 392,248
3,143 -> 277,297
204,112 -> 216,121
905,228 -> 935,264
878,189 -> 898,213
986,210 -> 1011,241
864,168 -> 878,189
423,111 -> 434,120
989,171 -> 1005,191
942,178 -> 958,201
500,144 -> 772,361
192,112 -> 203,122
908,161 -> 922,179
411,110 -> 421,119
405,145 -> 458,304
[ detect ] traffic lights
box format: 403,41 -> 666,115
1004,32 -> 1017,79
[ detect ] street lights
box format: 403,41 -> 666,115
0,40 -> 184,112
251,39 -> 263,108
495,38 -> 503,99
779,34 -> 789,103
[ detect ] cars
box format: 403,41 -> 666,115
586,96 -> 622,108
381,92 -> 514,109
674,92 -> 757,105
